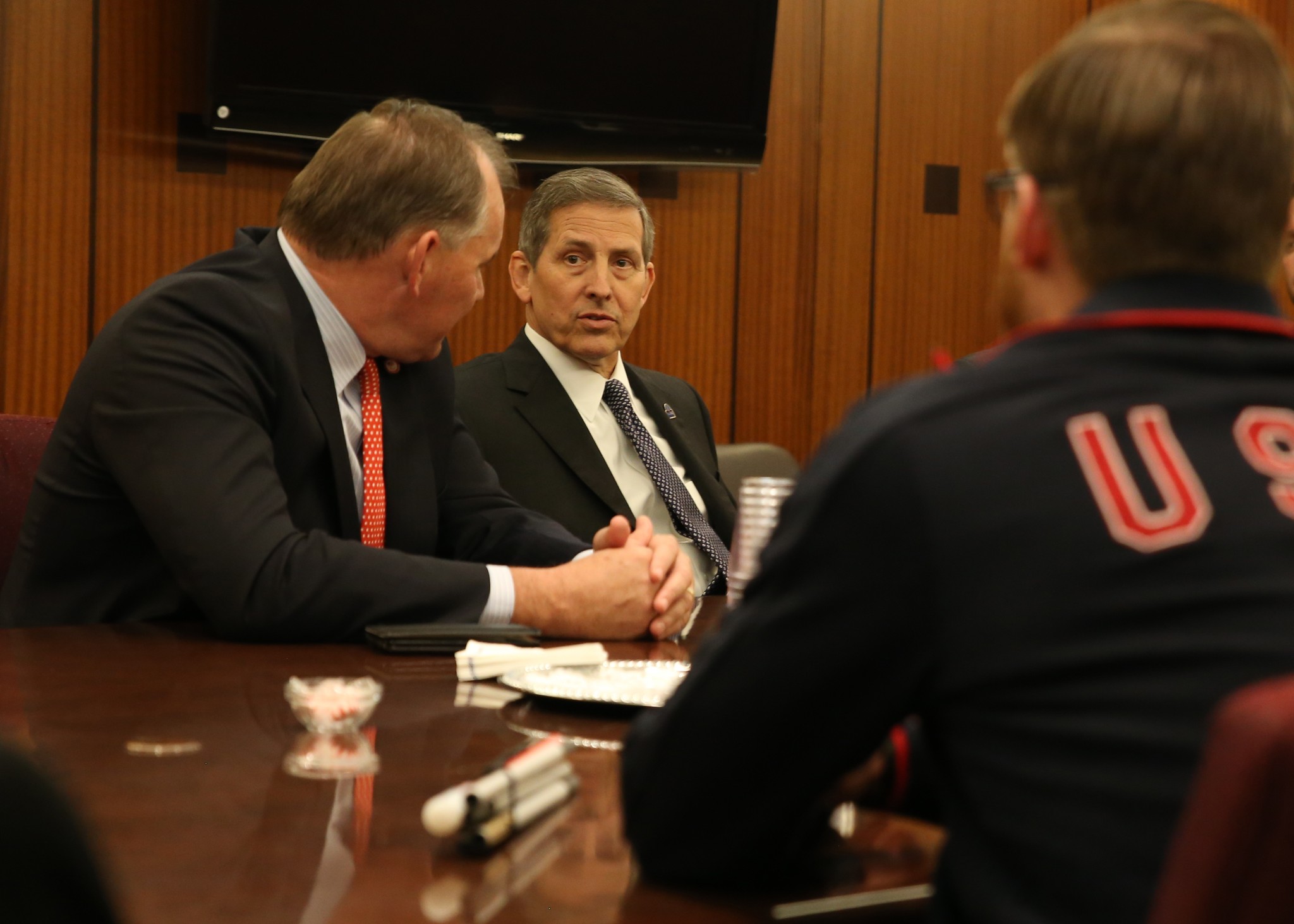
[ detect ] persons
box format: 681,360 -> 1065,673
450,169 -> 740,595
1,95 -> 704,648
616,0 -> 1294,924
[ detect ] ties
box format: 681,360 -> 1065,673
601,379 -> 732,598
359,355 -> 386,550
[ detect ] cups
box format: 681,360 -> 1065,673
726,476 -> 796,616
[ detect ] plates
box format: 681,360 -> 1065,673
496,657 -> 693,708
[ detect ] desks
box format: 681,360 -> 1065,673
0,588 -> 967,924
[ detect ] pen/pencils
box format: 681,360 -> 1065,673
418,730 -> 583,854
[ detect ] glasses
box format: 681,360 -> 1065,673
982,161 -> 1060,230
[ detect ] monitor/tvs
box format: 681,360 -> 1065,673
179,1 -> 781,173
